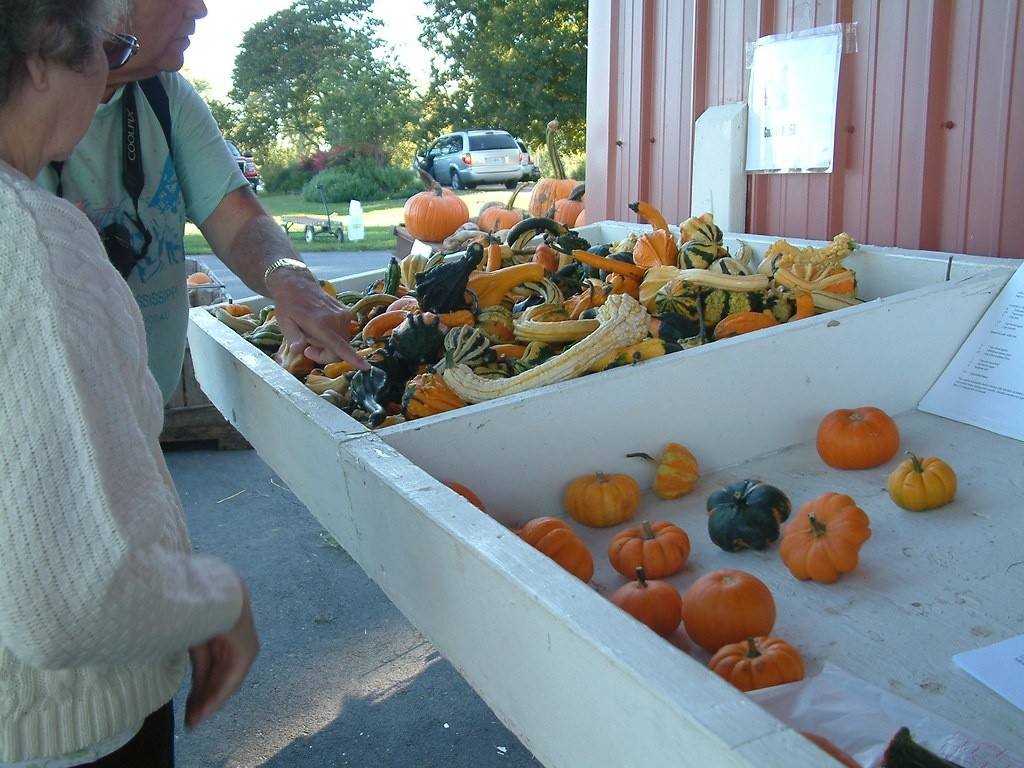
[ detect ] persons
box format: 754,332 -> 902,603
0,0 -> 262,768
32,1 -> 371,412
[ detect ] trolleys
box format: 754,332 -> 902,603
275,185 -> 346,246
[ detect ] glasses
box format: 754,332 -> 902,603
96,29 -> 139,68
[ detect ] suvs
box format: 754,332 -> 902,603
515,138 -> 535,178
226,141 -> 259,193
410,129 -> 525,192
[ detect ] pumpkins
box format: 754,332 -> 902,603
186,118 -> 973,768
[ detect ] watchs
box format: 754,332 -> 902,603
264,257 -> 314,293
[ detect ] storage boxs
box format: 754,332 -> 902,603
155,253 -> 253,449
